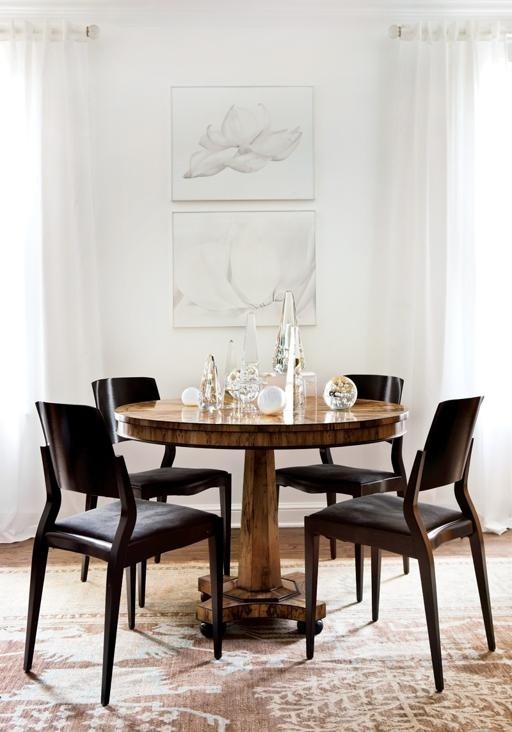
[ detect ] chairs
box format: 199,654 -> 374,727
78,377 -> 232,631
275,373 -> 410,604
23,401 -> 224,706
303,395 -> 495,694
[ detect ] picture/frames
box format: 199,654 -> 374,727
168,84 -> 314,202
170,210 -> 318,329
